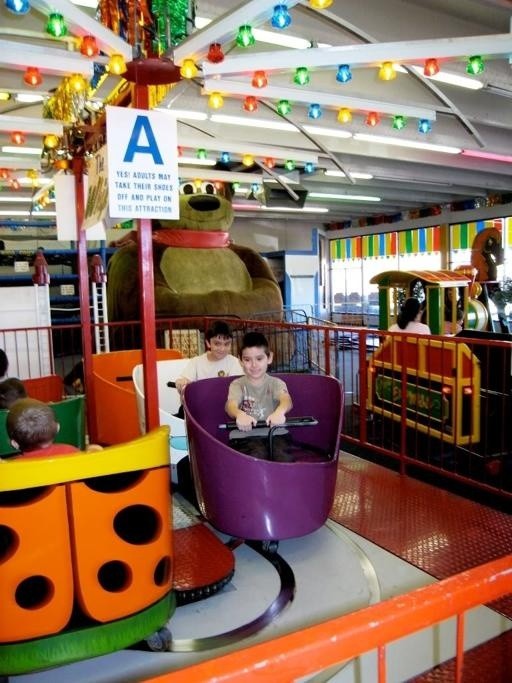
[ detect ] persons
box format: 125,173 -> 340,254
223,330 -> 295,465
389,297 -> 430,336
0,376 -> 28,407
6,397 -> 83,459
176,322 -> 244,399
447,286 -> 452,300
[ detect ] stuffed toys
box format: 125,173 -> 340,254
107,178 -> 298,370
